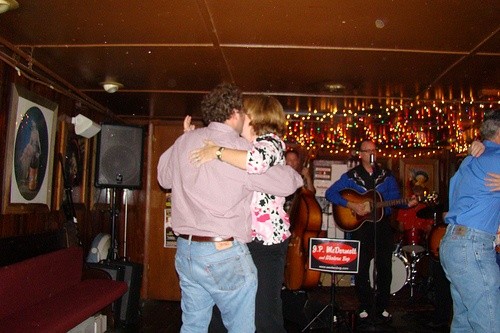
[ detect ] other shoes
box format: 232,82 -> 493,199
378,309 -> 393,321
357,306 -> 369,322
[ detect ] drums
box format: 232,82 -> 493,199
400,229 -> 427,254
368,251 -> 411,296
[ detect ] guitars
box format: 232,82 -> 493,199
332,188 -> 439,232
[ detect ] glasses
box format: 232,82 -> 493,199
360,149 -> 375,154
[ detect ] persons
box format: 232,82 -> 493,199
183,94 -> 291,333
157,82 -> 304,333
470,141 -> 500,266
439,109 -> 500,333
284,149 -> 317,195
325,139 -> 418,318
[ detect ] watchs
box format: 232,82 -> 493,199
216,146 -> 225,161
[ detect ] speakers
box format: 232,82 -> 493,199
94,121 -> 144,189
90,260 -> 143,325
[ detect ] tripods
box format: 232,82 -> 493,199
300,274 -> 351,333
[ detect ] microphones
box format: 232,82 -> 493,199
370,153 -> 374,165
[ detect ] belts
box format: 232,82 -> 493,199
179,234 -> 233,243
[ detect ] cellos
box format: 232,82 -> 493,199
283,143 -> 323,290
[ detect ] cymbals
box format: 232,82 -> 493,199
416,203 -> 448,219
